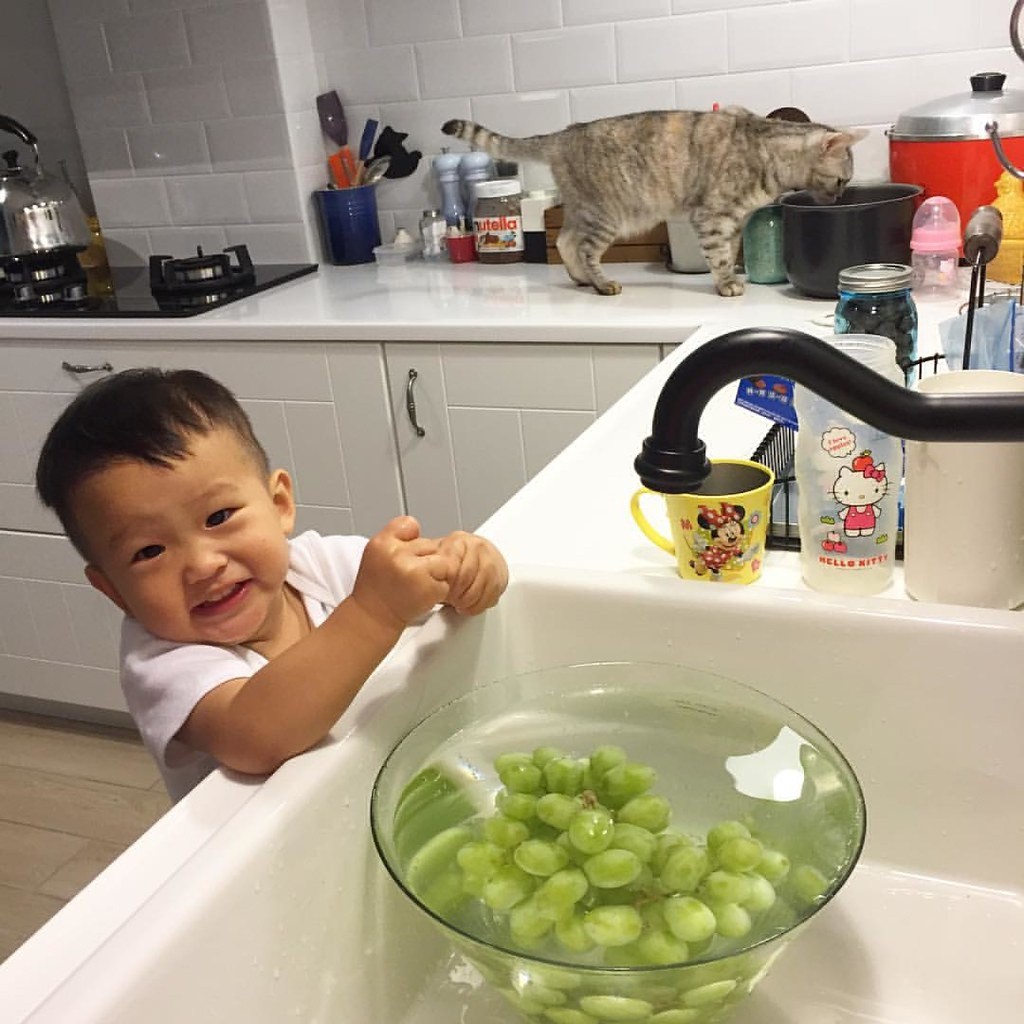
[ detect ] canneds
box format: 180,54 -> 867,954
834,263 -> 919,383
742,203 -> 790,284
472,179 -> 524,264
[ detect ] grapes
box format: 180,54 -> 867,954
457,744 -> 829,1024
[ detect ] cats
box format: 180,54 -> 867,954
439,109 -> 872,297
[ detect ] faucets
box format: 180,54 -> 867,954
631,325 -> 1023,496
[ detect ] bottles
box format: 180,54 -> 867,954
986,170 -> 1024,285
834,262 -> 918,389
793,334 -> 904,594
742,203 -> 785,284
395,227 -> 412,244
419,145 -> 554,261
910,197 -> 963,301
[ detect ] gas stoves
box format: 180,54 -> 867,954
0,254 -> 319,319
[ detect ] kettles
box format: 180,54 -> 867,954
0,113 -> 91,263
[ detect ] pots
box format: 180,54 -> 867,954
884,72 -> 1024,259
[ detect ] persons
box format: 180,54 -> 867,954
35,367 -> 509,805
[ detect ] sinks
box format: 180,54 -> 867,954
88,578 -> 1024,1024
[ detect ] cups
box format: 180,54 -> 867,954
630,458 -> 775,585
666,212 -> 705,270
313,185 -> 382,266
904,368 -> 1024,610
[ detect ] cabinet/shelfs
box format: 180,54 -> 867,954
0,338 -> 686,747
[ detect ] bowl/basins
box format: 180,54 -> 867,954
372,242 -> 422,266
368,661 -> 867,1024
777,182 -> 924,298
